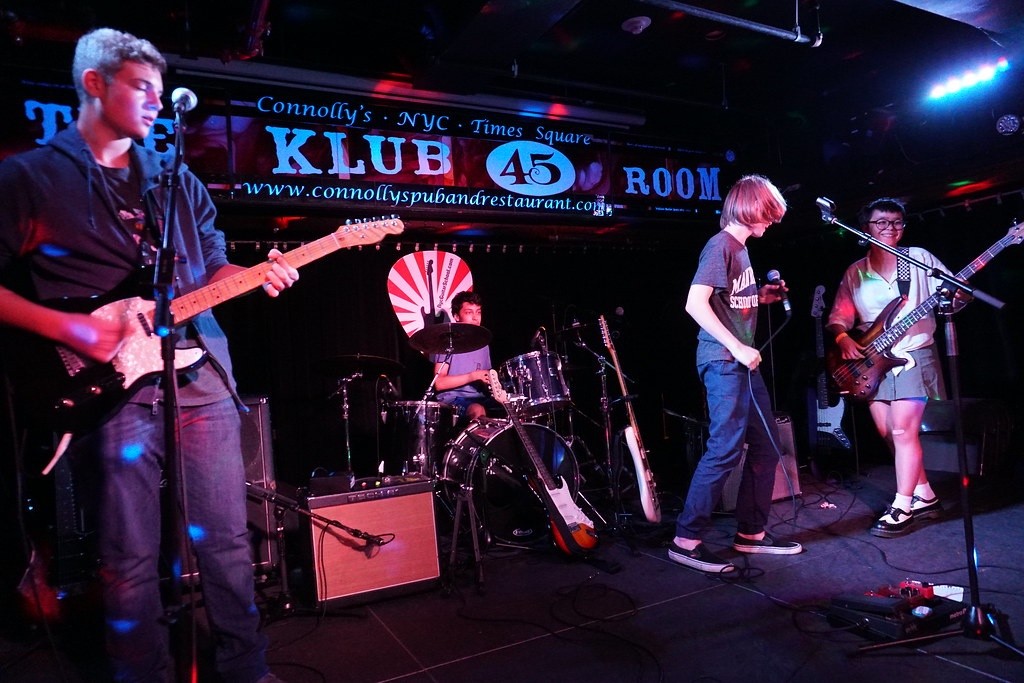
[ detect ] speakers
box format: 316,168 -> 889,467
296,471 -> 440,603
49,398 -> 280,587
712,415 -> 802,515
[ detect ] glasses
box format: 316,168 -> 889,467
865,217 -> 906,230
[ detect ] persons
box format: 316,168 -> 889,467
827,195 -> 976,539
434,291 -> 515,419
0,28 -> 301,683
668,174 -> 805,575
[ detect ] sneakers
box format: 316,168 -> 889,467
869,504 -> 915,537
668,541 -> 733,573
734,533 -> 802,556
910,494 -> 942,517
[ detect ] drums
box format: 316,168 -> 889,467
383,400 -> 461,483
438,417 -> 580,547
498,350 -> 572,420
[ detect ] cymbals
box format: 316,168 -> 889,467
409,322 -> 490,354
332,354 -> 401,378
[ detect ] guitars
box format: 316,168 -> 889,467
17,431 -> 105,626
9,214 -> 403,434
805,284 -> 851,480
826,220 -> 1024,396
598,314 -> 663,523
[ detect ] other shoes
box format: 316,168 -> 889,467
255,670 -> 283,682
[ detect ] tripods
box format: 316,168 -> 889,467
815,195 -> 1024,661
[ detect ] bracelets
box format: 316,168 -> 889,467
834,329 -> 848,346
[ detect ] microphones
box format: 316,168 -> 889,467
529,328 -> 542,350
385,376 -> 400,396
172,88 -> 198,113
768,269 -> 791,316
